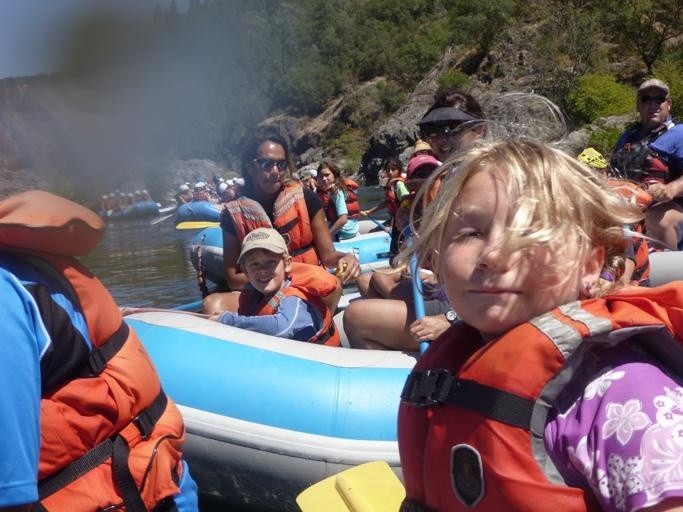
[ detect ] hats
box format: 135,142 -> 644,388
637,78 -> 670,93
415,108 -> 482,125
236,228 -> 289,263
412,140 -> 433,155
407,155 -> 443,180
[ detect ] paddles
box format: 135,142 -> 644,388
176,222 -> 220,230
295,461 -> 406,512
159,205 -> 177,213
150,214 -> 175,226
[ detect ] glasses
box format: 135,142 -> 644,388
255,155 -> 289,171
424,122 -> 465,138
640,95 -> 668,104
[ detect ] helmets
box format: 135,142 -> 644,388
575,147 -> 608,169
179,177 -> 245,193
102,189 -> 148,201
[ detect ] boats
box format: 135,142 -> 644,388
103,242 -> 683,503
189,219 -> 396,289
99,197 -> 162,225
176,199 -> 226,224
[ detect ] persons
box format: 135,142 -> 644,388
391,136 -> 683,512
174,133 -> 361,350
343,92 -> 488,350
2,189 -> 201,511
99,188 -> 151,211
599,178 -> 651,287
607,77 -> 683,251
